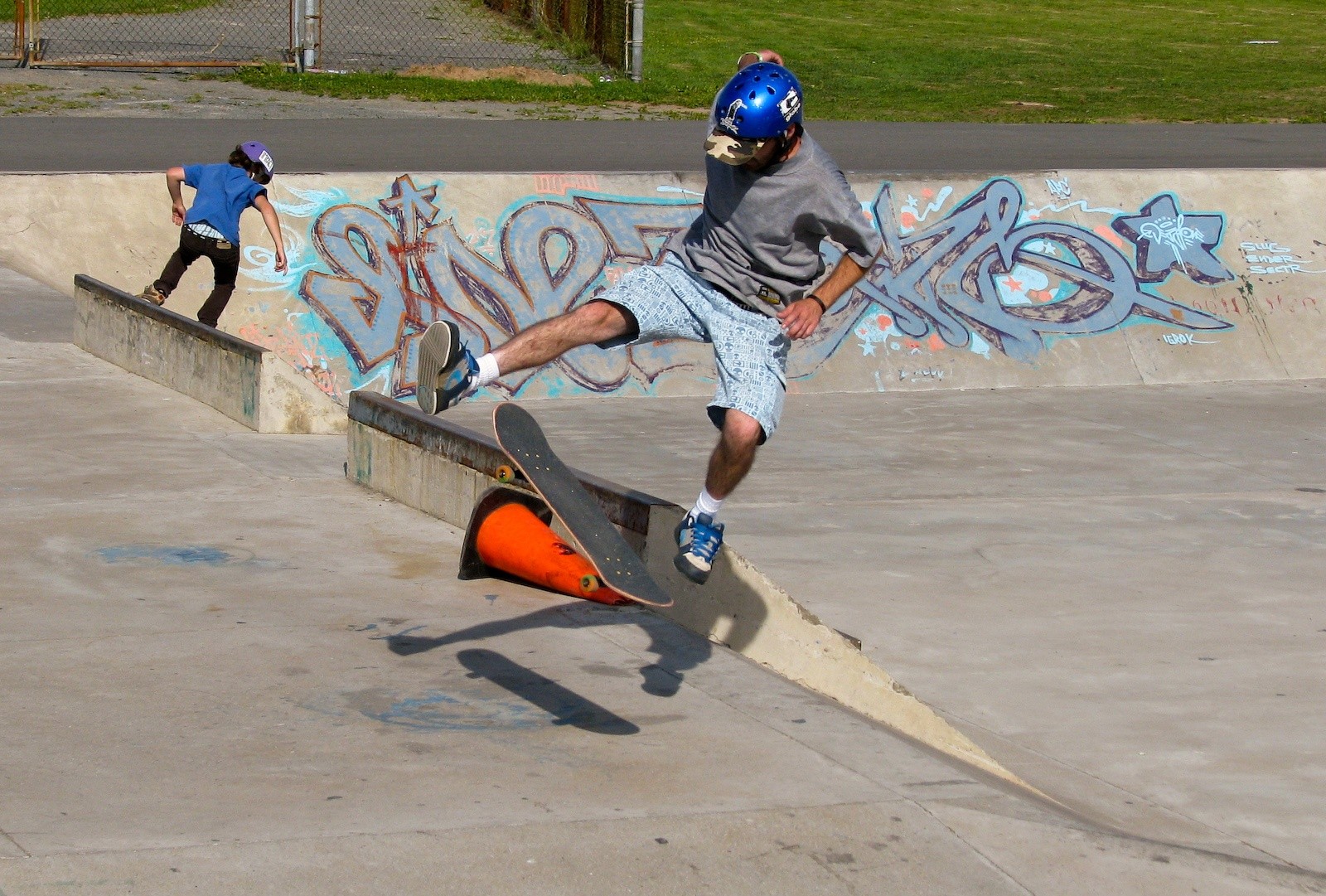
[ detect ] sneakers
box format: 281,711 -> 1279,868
417,321 -> 479,415
674,510 -> 725,584
136,284 -> 165,306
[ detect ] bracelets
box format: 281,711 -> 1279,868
737,51 -> 763,65
807,295 -> 826,315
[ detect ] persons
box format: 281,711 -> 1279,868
142,140 -> 288,328
416,49 -> 884,586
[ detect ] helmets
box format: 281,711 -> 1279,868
240,140 -> 274,185
710,61 -> 803,136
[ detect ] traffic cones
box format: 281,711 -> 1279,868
457,481 -> 639,607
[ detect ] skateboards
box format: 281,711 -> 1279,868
491,402 -> 675,609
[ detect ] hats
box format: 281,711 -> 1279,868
703,125 -> 766,166
